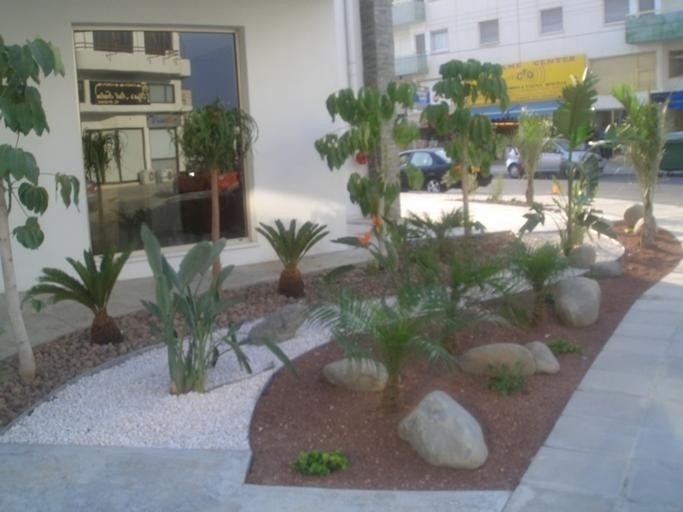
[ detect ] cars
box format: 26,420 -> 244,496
501,134 -> 606,180
163,165 -> 243,194
398,146 -> 494,192
85,174 -> 99,211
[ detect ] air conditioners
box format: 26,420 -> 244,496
137,168 -> 173,186
